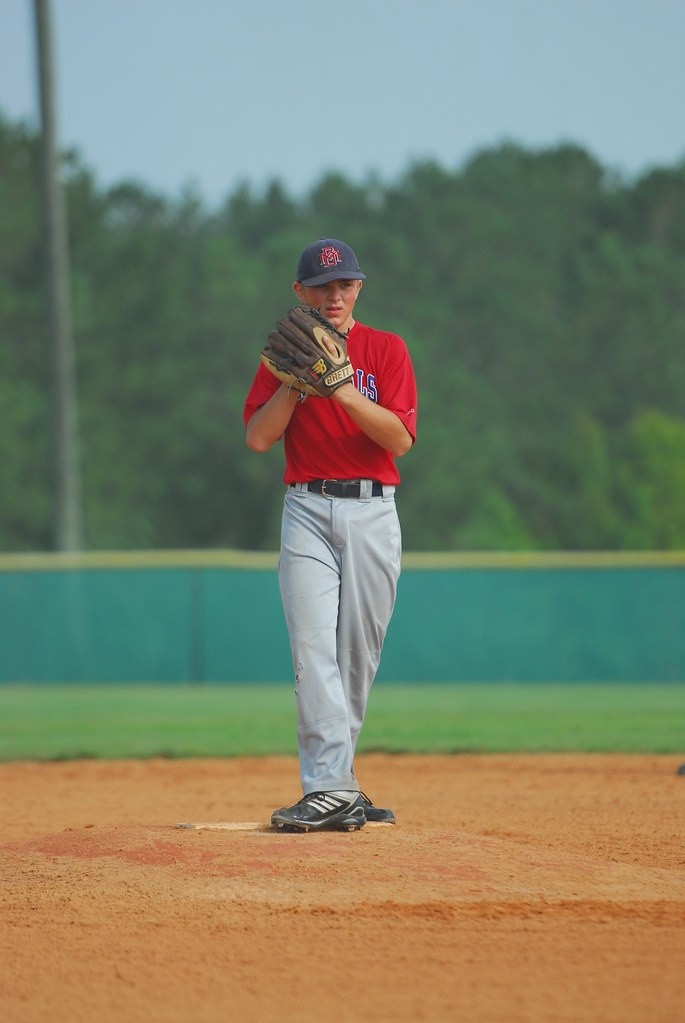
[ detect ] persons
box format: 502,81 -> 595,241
239,237 -> 416,831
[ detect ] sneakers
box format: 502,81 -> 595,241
360,794 -> 396,824
270,791 -> 367,832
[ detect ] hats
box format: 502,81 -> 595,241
296,237 -> 367,287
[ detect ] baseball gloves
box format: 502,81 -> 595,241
260,304 -> 356,398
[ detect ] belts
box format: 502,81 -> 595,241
290,479 -> 382,498
